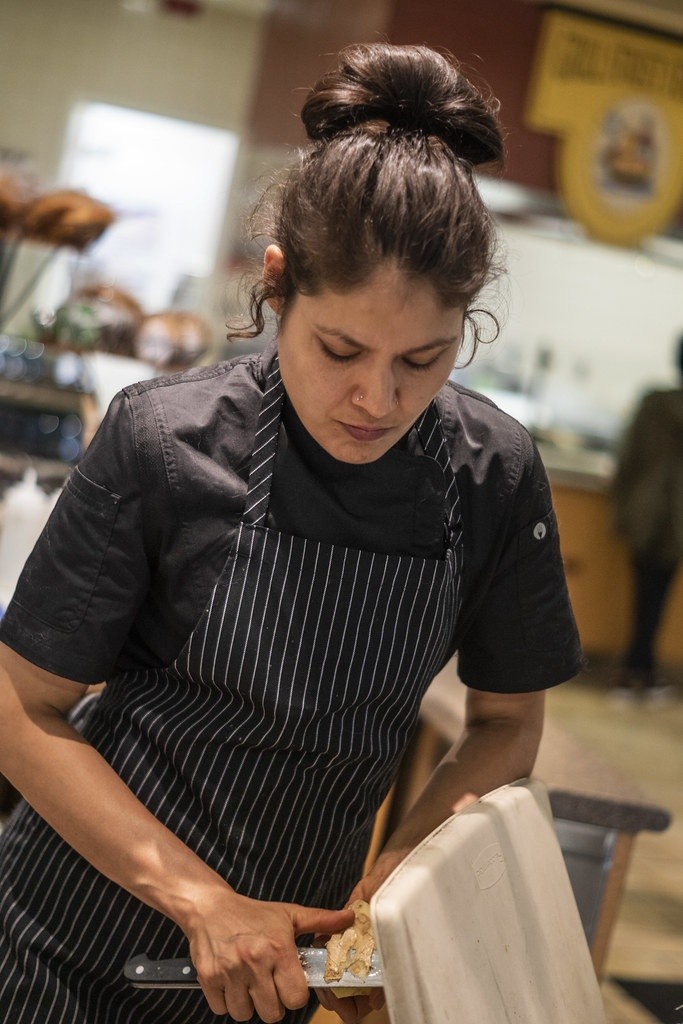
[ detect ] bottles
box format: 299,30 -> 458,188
1,465 -> 49,611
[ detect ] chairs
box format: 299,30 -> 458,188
547,787 -> 673,995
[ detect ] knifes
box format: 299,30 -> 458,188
123,947 -> 385,989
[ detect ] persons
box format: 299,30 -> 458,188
0,45 -> 583,1024
608,335 -> 683,686
